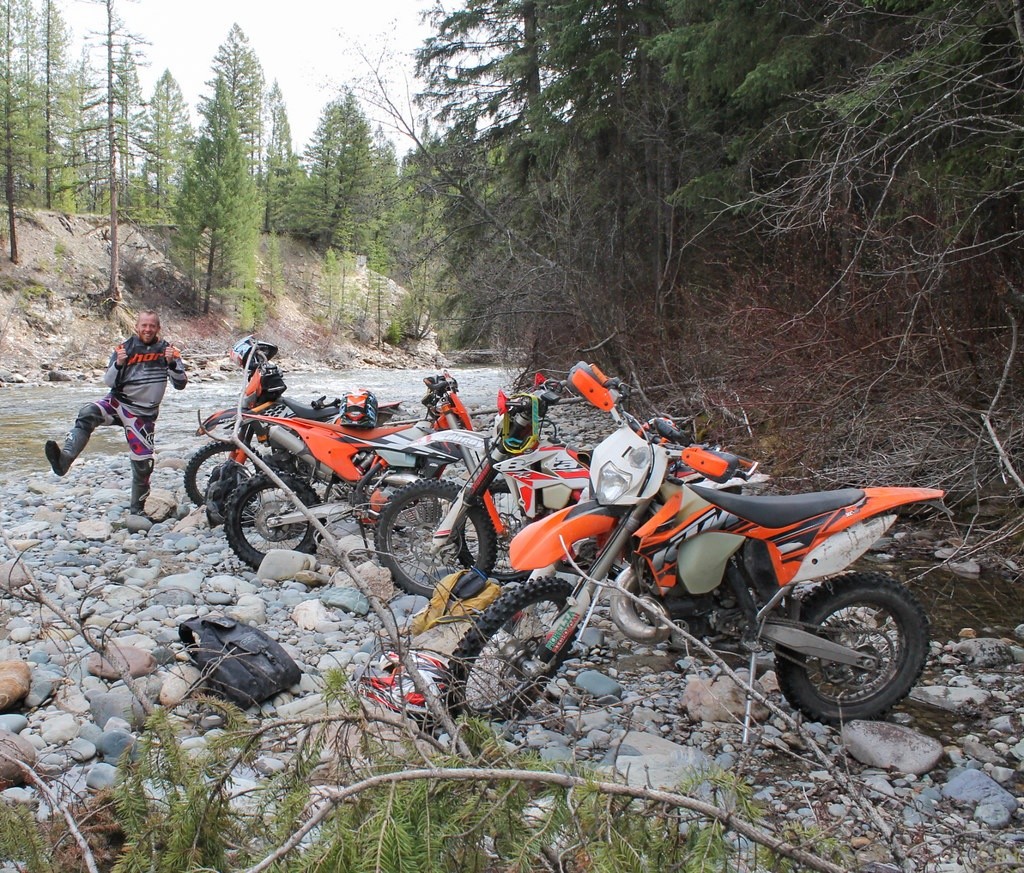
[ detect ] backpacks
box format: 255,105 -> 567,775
179,615 -> 302,708
205,459 -> 256,529
412,569 -> 501,635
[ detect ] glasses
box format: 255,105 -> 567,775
503,436 -> 536,455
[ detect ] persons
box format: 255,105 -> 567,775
45,310 -> 187,521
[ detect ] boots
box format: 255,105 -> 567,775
131,480 -> 154,521
45,428 -> 90,476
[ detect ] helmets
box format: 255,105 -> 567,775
230,335 -> 278,369
339,389 -> 378,428
361,649 -> 451,729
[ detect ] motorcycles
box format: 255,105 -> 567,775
182,327 -> 777,604
433,359 -> 958,730
